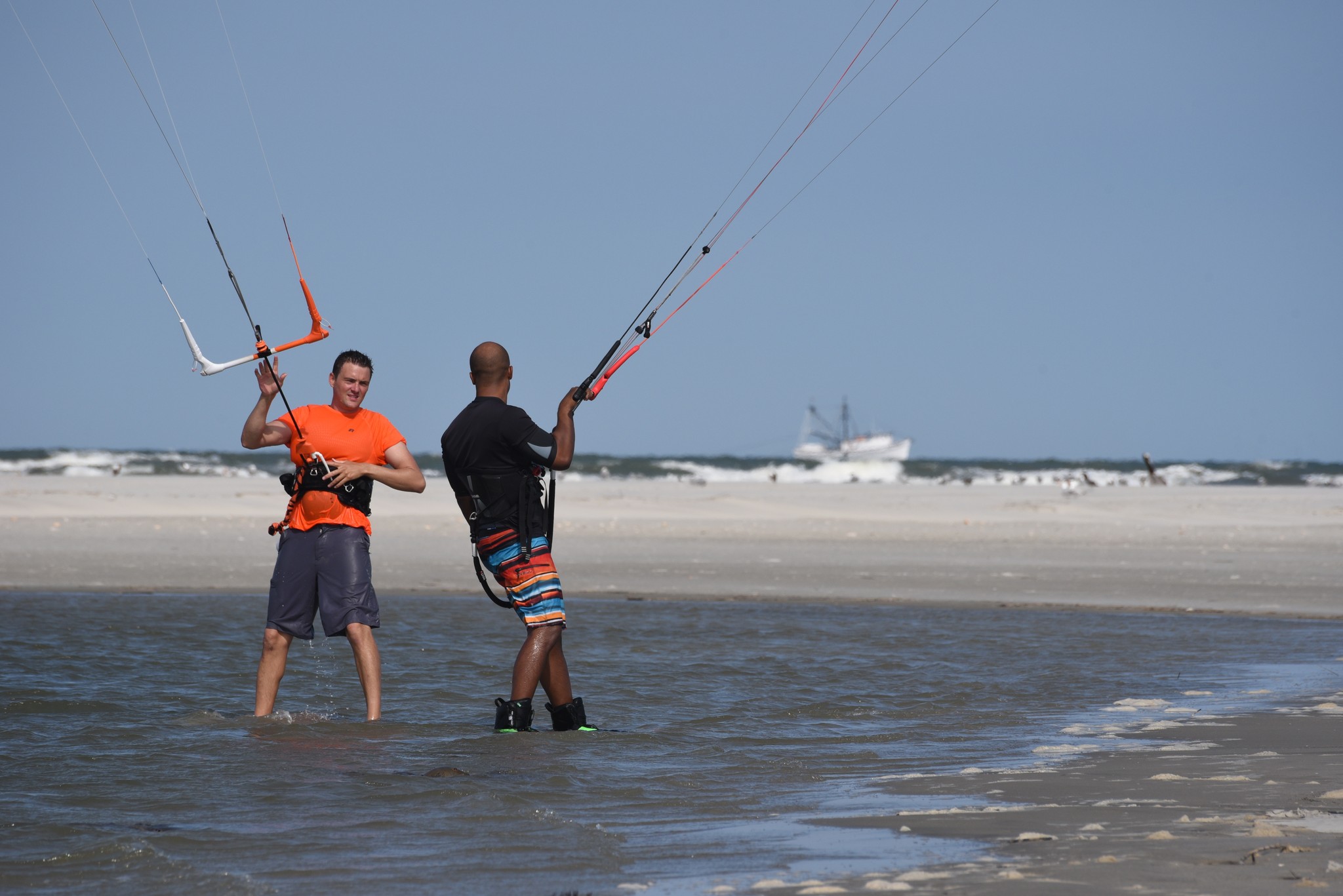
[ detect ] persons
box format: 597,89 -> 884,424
239,350 -> 427,720
439,341 -> 598,733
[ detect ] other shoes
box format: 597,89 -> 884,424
493,697 -> 538,733
544,697 -> 600,730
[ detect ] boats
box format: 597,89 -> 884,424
795,399 -> 915,466
